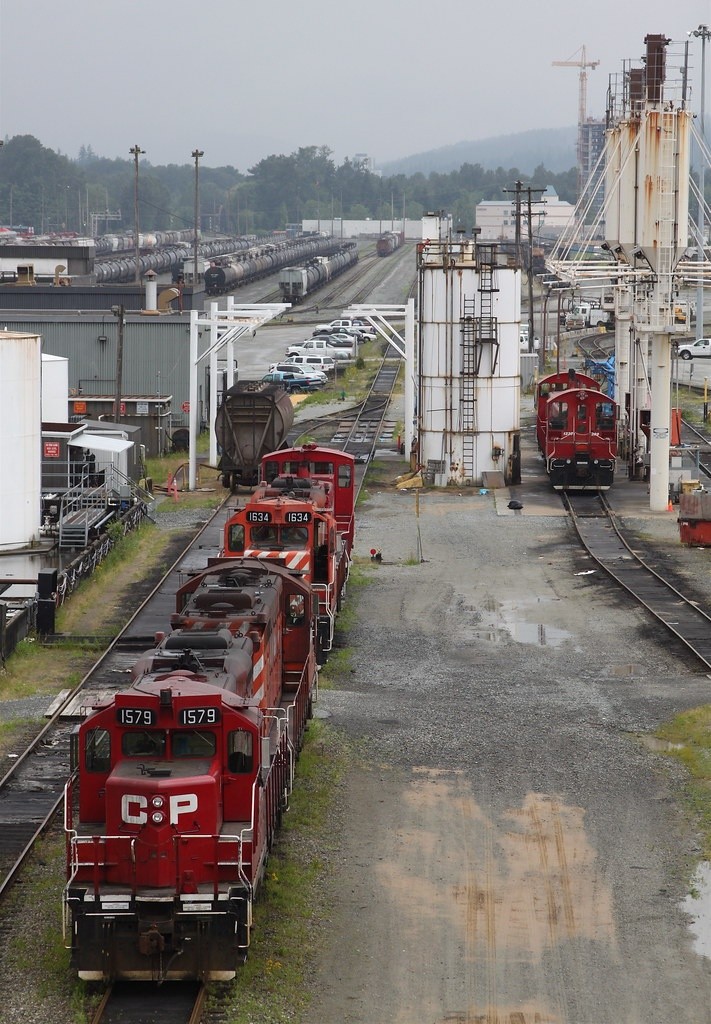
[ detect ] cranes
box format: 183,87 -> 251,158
550,44 -> 601,125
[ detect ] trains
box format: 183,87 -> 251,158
535,362 -> 626,496
61,434 -> 356,988
10,224 -> 404,305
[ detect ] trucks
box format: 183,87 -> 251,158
566,304 -> 611,328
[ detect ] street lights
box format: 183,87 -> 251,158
188,149 -> 203,280
127,143 -> 147,285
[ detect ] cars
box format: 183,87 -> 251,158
263,316 -> 378,394
675,336 -> 707,363
